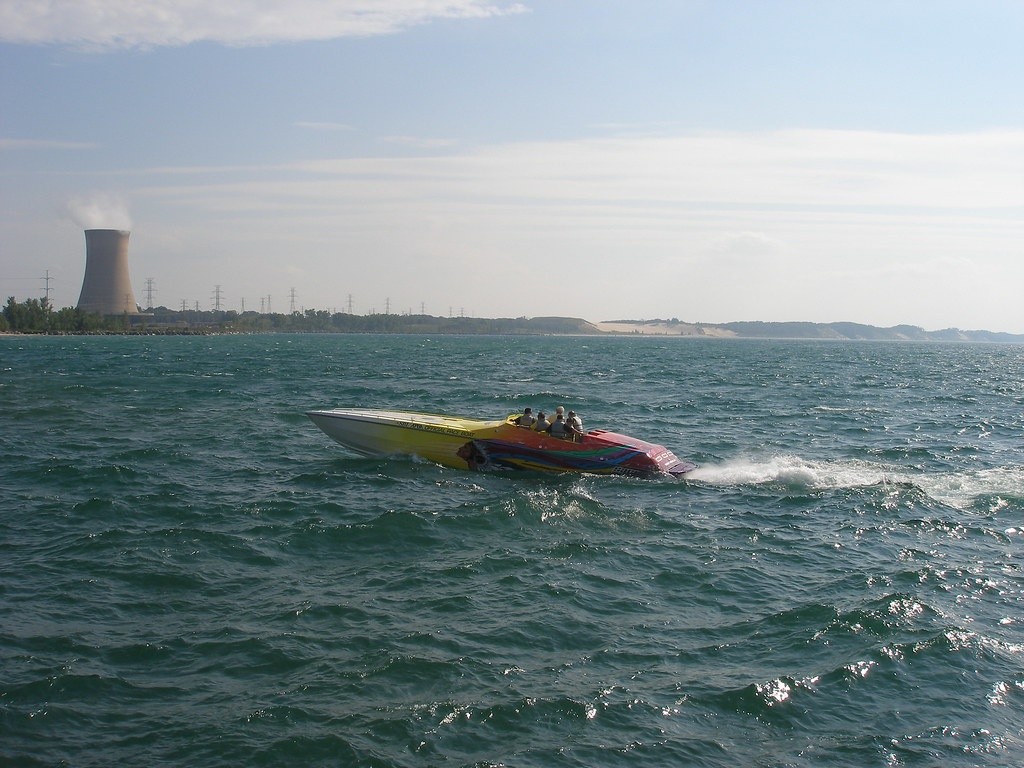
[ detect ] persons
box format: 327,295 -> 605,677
529,406 -> 583,439
514,408 -> 538,426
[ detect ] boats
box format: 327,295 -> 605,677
303,408 -> 699,480
